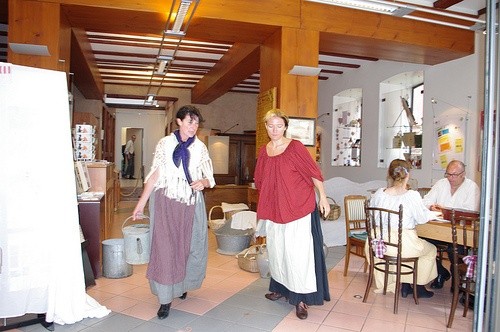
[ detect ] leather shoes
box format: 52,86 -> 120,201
430,270 -> 450,289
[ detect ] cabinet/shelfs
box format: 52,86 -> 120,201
71,97 -> 120,279
214,132 -> 256,186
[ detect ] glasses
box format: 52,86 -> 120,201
444,171 -> 464,178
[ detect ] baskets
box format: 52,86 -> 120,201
317,196 -> 341,221
238,245 -> 260,273
209,206 -> 226,230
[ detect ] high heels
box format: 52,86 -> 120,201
401,288 -> 413,297
296,301 -> 308,320
265,292 -> 289,302
413,287 -> 434,298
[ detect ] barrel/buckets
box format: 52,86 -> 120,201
121,214 -> 151,265
214,224 -> 254,255
102,238 -> 133,279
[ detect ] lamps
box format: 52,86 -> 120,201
164,0 -> 193,36
152,55 -> 174,76
145,94 -> 155,103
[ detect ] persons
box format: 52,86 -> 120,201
371,159 -> 438,298
134,106 -> 215,319
254,108 -> 330,319
423,160 -> 481,294
122,135 -> 137,179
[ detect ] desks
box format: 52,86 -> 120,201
416,219 -> 480,249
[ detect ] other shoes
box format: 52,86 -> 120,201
157,301 -> 172,319
178,291 -> 187,299
129,176 -> 136,179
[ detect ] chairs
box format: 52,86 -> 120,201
344,195 -> 368,276
364,200 -> 420,314
446,209 -> 478,328
